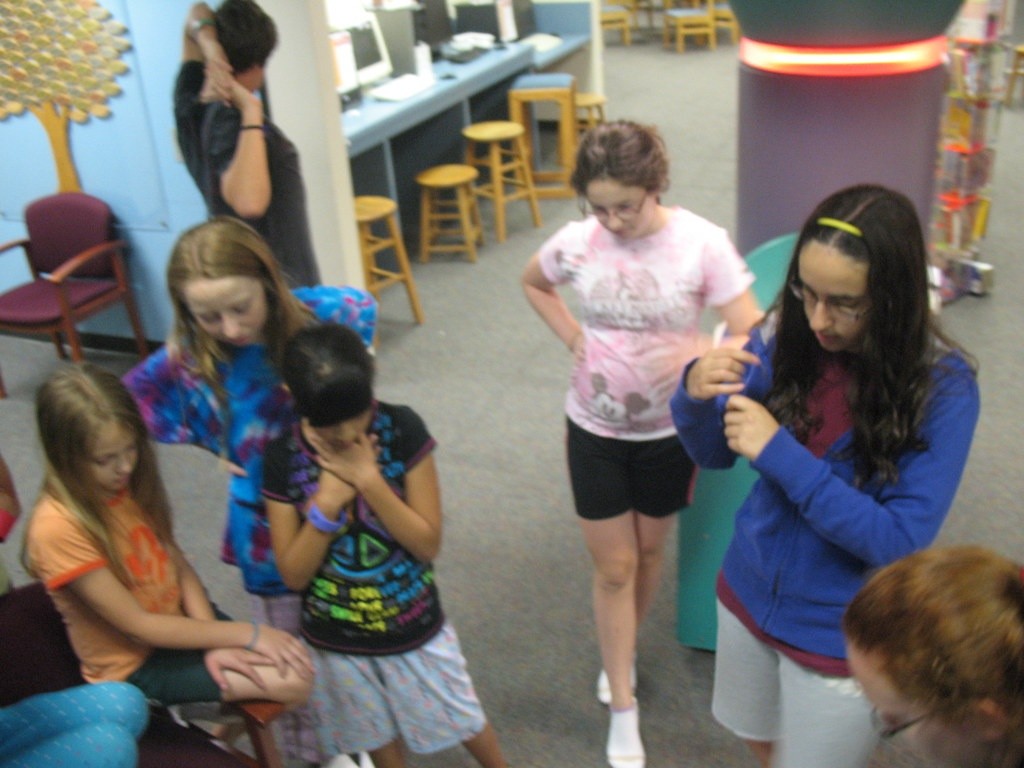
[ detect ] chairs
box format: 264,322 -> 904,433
0,578 -> 285,768
598,0 -> 739,55
0,193 -> 149,402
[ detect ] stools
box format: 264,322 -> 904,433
416,162 -> 485,265
353,194 -> 424,350
570,90 -> 606,133
461,119 -> 547,246
505,74 -> 577,203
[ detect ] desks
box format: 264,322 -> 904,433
339,39 -> 532,241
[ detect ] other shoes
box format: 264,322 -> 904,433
597,658 -> 635,704
607,696 -> 646,768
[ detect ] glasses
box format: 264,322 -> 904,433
789,272 -> 873,323
582,186 -> 646,220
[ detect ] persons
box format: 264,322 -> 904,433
0,453 -> 148,768
842,547 -> 1023,767
119,215 -> 379,768
669,184 -> 980,768
18,363 -> 315,744
521,120 -> 759,768
260,324 -> 510,768
174,0 -> 322,290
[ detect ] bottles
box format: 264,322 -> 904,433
413,40 -> 434,87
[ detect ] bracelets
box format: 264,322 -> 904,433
192,19 -> 213,35
238,125 -> 265,129
304,500 -> 349,535
246,621 -> 259,653
